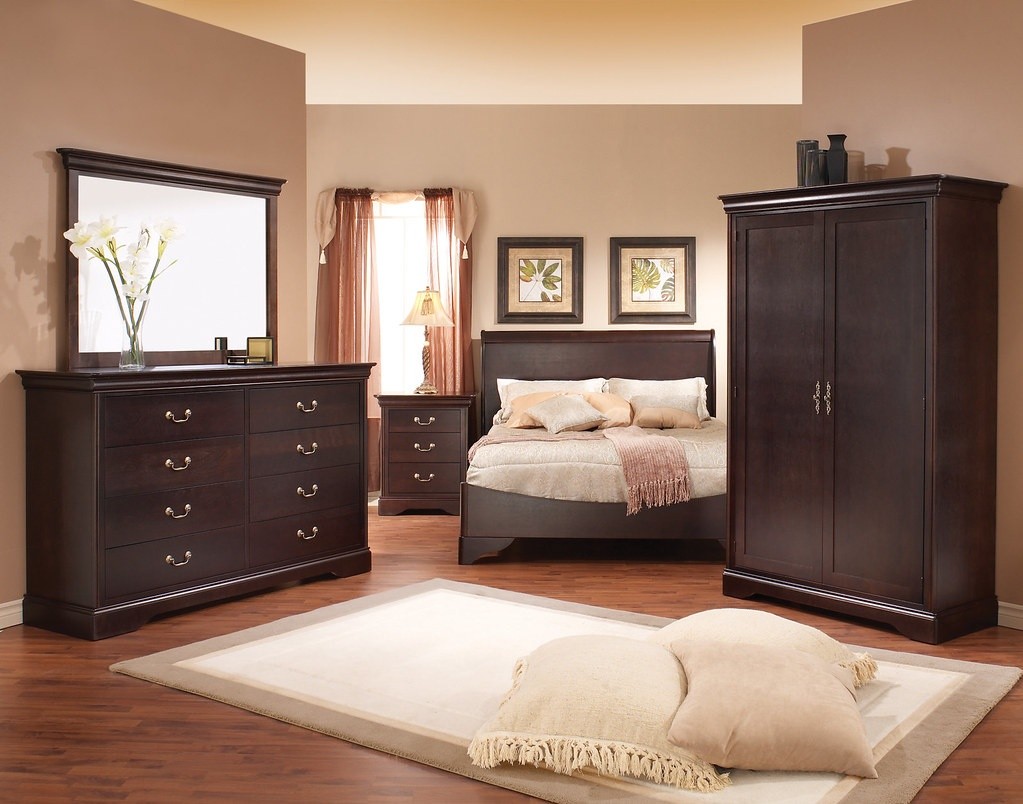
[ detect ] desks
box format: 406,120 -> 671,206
15,362 -> 377,643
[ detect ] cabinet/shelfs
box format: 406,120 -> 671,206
715,171 -> 1010,645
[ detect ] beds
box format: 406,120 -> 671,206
457,329 -> 728,565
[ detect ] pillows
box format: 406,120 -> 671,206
496,377 -> 711,434
470,607 -> 881,791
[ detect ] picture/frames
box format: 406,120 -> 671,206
608,237 -> 697,325
495,235 -> 584,324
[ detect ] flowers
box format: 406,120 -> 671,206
65,211 -> 190,354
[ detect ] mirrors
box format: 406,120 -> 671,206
55,146 -> 288,366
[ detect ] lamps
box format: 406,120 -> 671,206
399,287 -> 455,396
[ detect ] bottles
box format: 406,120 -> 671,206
826,134 -> 848,184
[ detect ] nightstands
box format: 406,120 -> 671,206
373,393 -> 478,517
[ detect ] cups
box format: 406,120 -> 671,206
796,140 -> 819,186
805,150 -> 827,186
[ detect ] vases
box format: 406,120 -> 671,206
113,297 -> 154,371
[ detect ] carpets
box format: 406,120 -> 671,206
108,575 -> 1023,804
